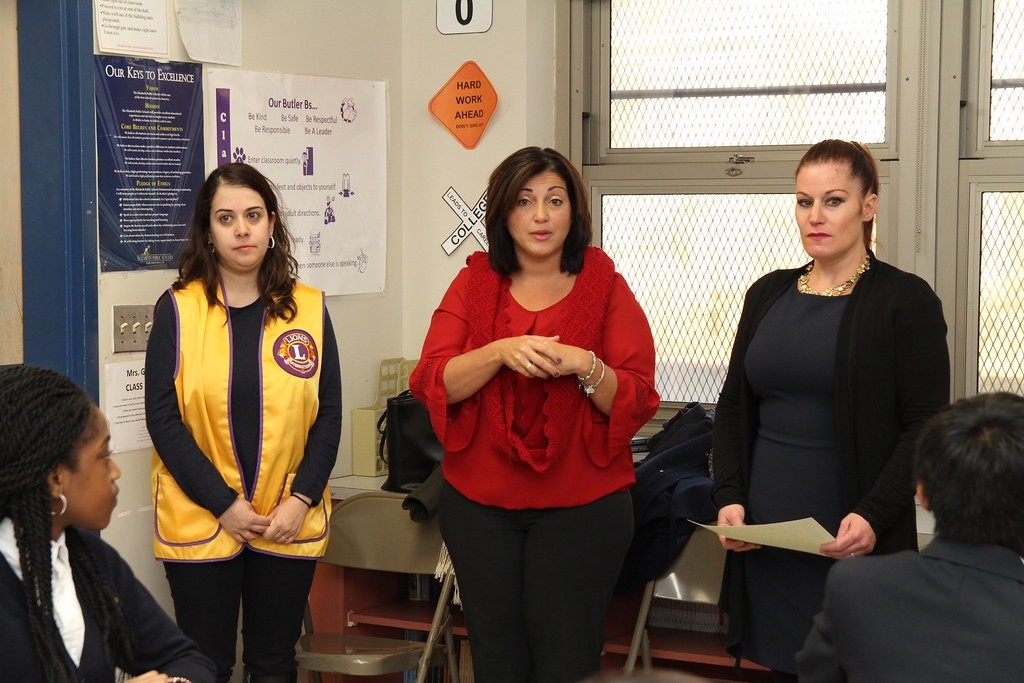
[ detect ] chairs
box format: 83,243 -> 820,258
621,522 -> 728,683
294,490 -> 463,683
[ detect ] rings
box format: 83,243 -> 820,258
526,363 -> 532,369
851,553 -> 854,557
282,536 -> 288,541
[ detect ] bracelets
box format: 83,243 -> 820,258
290,494 -> 311,508
577,351 -> 596,380
582,358 -> 605,397
169,677 -> 191,683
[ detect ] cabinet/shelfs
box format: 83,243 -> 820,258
307,474 -> 770,683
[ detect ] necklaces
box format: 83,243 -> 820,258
798,255 -> 870,296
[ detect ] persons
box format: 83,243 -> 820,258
714,140 -> 949,683
797,391 -> 1024,683
145,163 -> 343,683
409,146 -> 659,683
0,364 -> 215,683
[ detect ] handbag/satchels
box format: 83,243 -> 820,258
378,388 -> 444,493
